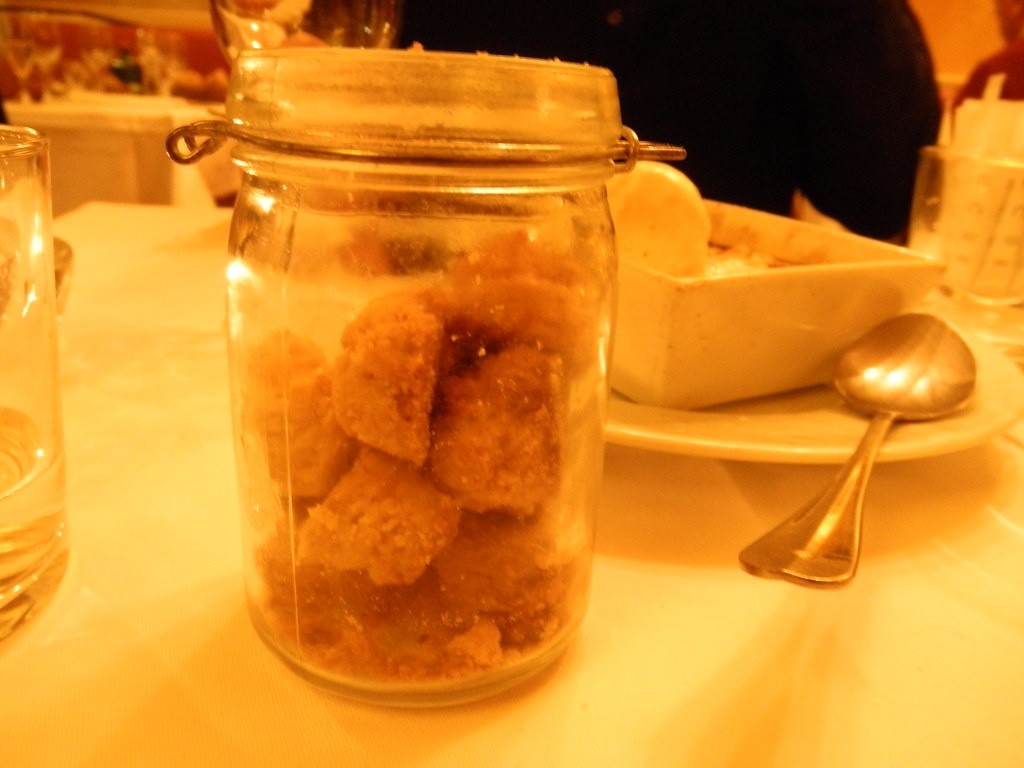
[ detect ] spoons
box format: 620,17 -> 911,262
741,313 -> 976,582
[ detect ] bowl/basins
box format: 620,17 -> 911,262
602,196 -> 945,408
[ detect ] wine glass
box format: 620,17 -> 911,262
2,5 -> 183,114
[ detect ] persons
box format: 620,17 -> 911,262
396,0 -> 944,241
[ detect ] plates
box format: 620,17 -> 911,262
594,318 -> 1024,462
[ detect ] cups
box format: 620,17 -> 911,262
909,149 -> 1024,426
0,124 -> 65,639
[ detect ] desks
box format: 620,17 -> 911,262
0,199 -> 1024,768
